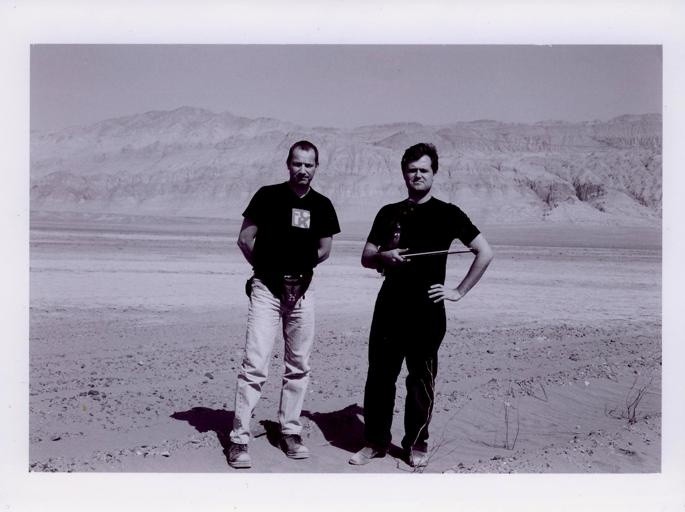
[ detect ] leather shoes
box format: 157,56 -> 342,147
278,434 -> 309,459
349,446 -> 428,467
226,443 -> 252,468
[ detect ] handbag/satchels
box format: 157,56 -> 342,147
280,275 -> 303,309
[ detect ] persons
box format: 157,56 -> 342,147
343,139 -> 492,466
226,137 -> 340,466
